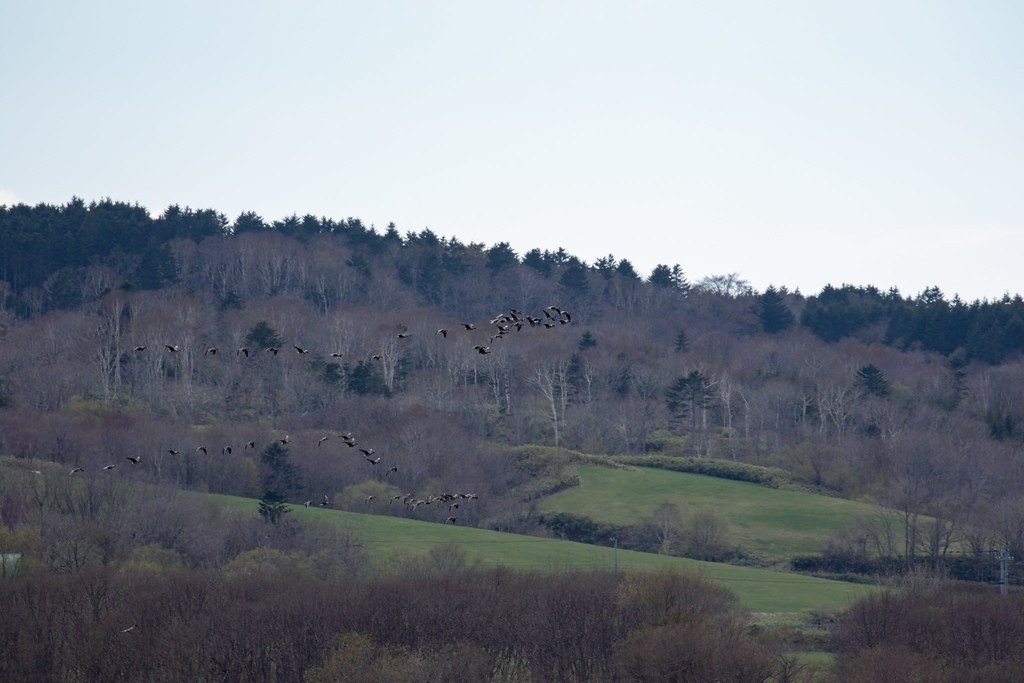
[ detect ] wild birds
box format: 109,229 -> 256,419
280,434 -> 292,444
459,305 -> 573,355
395,331 -> 408,339
204,348 -> 218,356
294,346 -> 309,354
167,449 -> 179,456
329,353 -> 343,357
69,467 -> 84,476
103,464 -> 115,470
372,354 -> 380,360
164,344 -> 181,352
222,446 -> 232,455
435,328 -> 449,339
195,445 -> 209,455
265,347 -> 280,355
243,441 -> 256,450
134,346 -> 146,352
126,455 -> 142,464
317,431 -> 397,476
237,348 -> 249,357
302,491 -> 481,526
